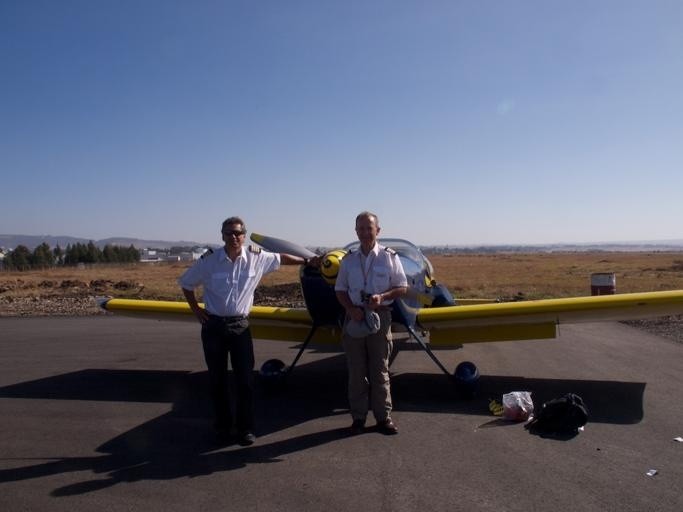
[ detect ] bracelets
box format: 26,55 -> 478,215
303,257 -> 308,265
381,294 -> 384,302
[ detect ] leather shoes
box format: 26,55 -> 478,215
377,419 -> 398,434
351,417 -> 366,434
241,433 -> 254,444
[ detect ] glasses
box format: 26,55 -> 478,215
222,229 -> 244,236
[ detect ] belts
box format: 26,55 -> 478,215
208,315 -> 241,324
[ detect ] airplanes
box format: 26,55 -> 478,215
100,232 -> 683,381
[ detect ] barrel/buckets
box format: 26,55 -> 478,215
590,272 -> 619,296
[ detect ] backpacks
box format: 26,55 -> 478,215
524,392 -> 589,440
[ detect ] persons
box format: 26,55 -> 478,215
179,217 -> 318,444
335,212 -> 409,434
